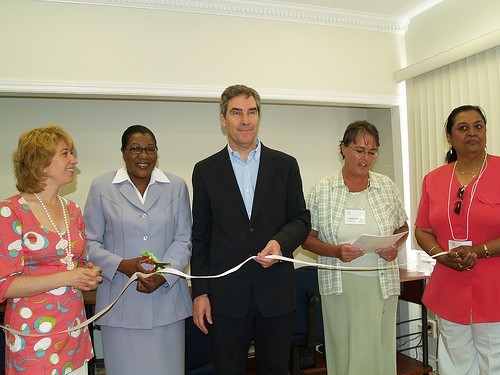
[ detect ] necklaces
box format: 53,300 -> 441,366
34,193 -> 77,294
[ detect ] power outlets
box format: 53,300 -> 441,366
427,319 -> 438,338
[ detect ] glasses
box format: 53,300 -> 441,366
346,145 -> 380,158
454,187 -> 465,216
123,144 -> 159,154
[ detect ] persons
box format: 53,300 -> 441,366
83,124 -> 193,375
0,125 -> 103,375
190,84 -> 312,375
415,105 -> 500,375
301,120 -> 409,375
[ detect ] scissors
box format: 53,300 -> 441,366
142,256 -> 170,272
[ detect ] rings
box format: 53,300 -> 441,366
348,258 -> 351,262
458,263 -> 463,267
466,267 -> 470,270
388,256 -> 391,260
456,252 -> 459,257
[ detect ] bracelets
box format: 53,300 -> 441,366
429,245 -> 439,254
483,242 -> 490,258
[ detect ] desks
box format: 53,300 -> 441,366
83,275 -> 432,367
